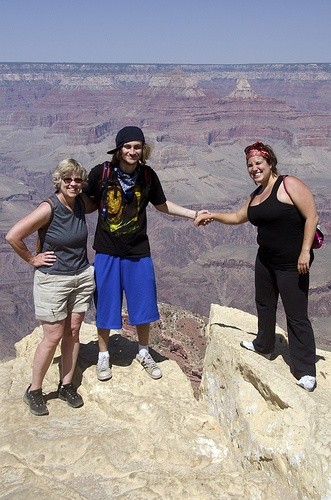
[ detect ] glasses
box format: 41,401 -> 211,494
62,178 -> 84,183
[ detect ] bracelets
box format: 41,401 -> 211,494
194,211 -> 198,220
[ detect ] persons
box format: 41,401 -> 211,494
80,126 -> 214,381
5,158 -> 95,415
194,141 -> 321,392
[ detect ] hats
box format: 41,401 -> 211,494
107,126 -> 145,154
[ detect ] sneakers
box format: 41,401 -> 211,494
297,375 -> 316,391
57,379 -> 84,407
136,352 -> 162,379
240,341 -> 271,359
96,355 -> 112,380
23,383 -> 49,416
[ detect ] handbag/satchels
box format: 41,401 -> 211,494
310,228 -> 324,249
